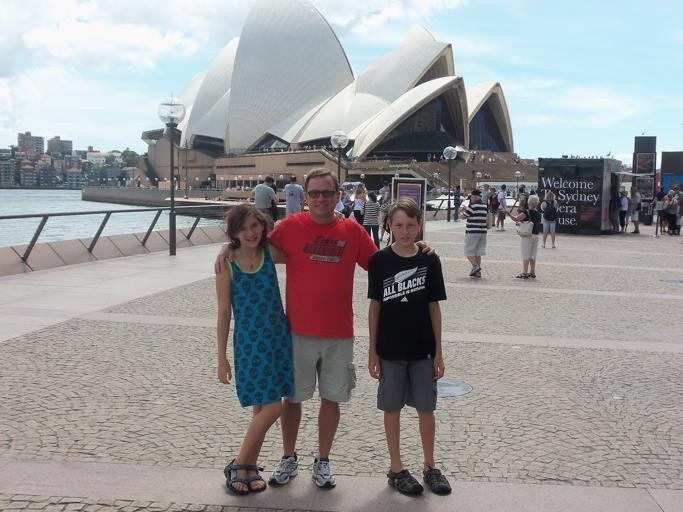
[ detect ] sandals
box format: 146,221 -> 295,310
516,271 -> 537,279
223,459 -> 248,494
246,463 -> 267,492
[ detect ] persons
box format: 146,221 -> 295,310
271,184 -> 277,221
611,183 -> 683,236
214,168 -> 440,490
250,176 -> 279,222
367,196 -> 453,496
336,181 -> 390,251
427,152 -> 559,280
214,202 -> 347,495
283,175 -> 306,218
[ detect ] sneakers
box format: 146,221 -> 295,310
312,456 -> 336,488
469,266 -> 482,277
386,466 -> 424,496
423,468 -> 452,495
267,451 -> 298,486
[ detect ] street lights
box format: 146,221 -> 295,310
329,126 -> 348,186
442,146 -> 458,221
514,170 -> 521,198
156,91 -> 190,257
125,173 -> 309,206
475,171 -> 482,188
359,173 -> 365,182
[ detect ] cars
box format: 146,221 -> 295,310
340,181 -> 365,202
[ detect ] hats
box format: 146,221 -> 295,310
619,190 -> 627,197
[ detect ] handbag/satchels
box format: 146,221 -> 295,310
636,202 -> 641,211
516,220 -> 534,238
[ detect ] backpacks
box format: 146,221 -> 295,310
491,191 -> 503,210
543,199 -> 556,222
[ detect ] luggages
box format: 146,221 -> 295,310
644,214 -> 652,226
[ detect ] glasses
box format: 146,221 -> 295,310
305,190 -> 337,197
